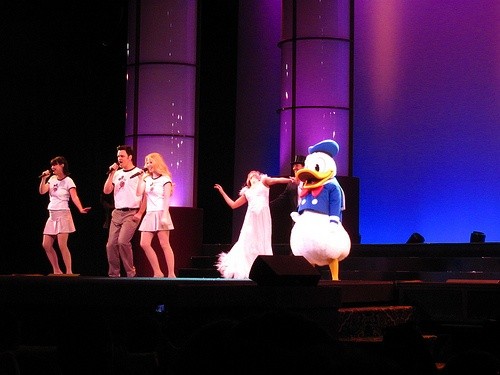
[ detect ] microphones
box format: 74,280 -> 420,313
107,162 -> 121,175
38,170 -> 54,178
130,168 -> 148,179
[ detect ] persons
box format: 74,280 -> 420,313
38,155 -> 93,275
212,169 -> 292,280
136,152 -> 176,280
0,289 -> 500,375
270,153 -> 306,217
103,144 -> 146,278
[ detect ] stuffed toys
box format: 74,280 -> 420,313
289,138 -> 351,280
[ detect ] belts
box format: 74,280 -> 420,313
116,208 -> 139,212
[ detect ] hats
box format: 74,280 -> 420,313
291,154 -> 307,164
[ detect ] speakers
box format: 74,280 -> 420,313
249,255 -> 321,286
